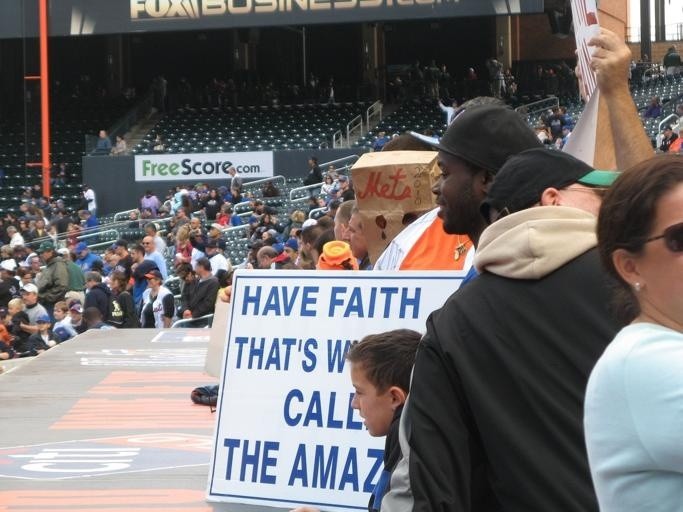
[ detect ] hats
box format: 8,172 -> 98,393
34,241 -> 56,252
479,146 -> 624,224
143,269 -> 162,280
72,241 -> 87,254
35,313 -> 51,322
409,96 -> 546,172
205,222 -> 222,232
19,283 -> 38,294
69,304 -> 83,313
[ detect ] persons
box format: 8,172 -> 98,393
175,263 -> 200,319
385,49 -> 579,135
186,258 -> 219,328
290,329 -> 420,512
69,303 -> 88,334
0,341 -> 14,360
636,54 -> 650,79
140,270 -> 174,329
431,106 -> 548,287
663,46 -> 681,76
382,26 -> 657,512
583,153 -> 683,511
105,167 -> 252,273
6,298 -> 31,350
0,184 -> 104,290
151,71 -> 336,110
111,135 -> 126,155
54,74 -> 136,105
253,155 -> 374,271
20,283 -> 49,335
52,302 -> 78,340
84,272 -> 113,325
375,130 -> 387,152
84,307 -> 116,331
107,270 -> 142,328
0,310 -> 15,346
27,314 -> 56,354
643,88 -> 682,151
95,128 -> 112,156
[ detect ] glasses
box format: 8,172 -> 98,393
636,220 -> 683,254
141,242 -> 150,245
145,276 -> 155,280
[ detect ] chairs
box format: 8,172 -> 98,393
0,72 -> 683,331
